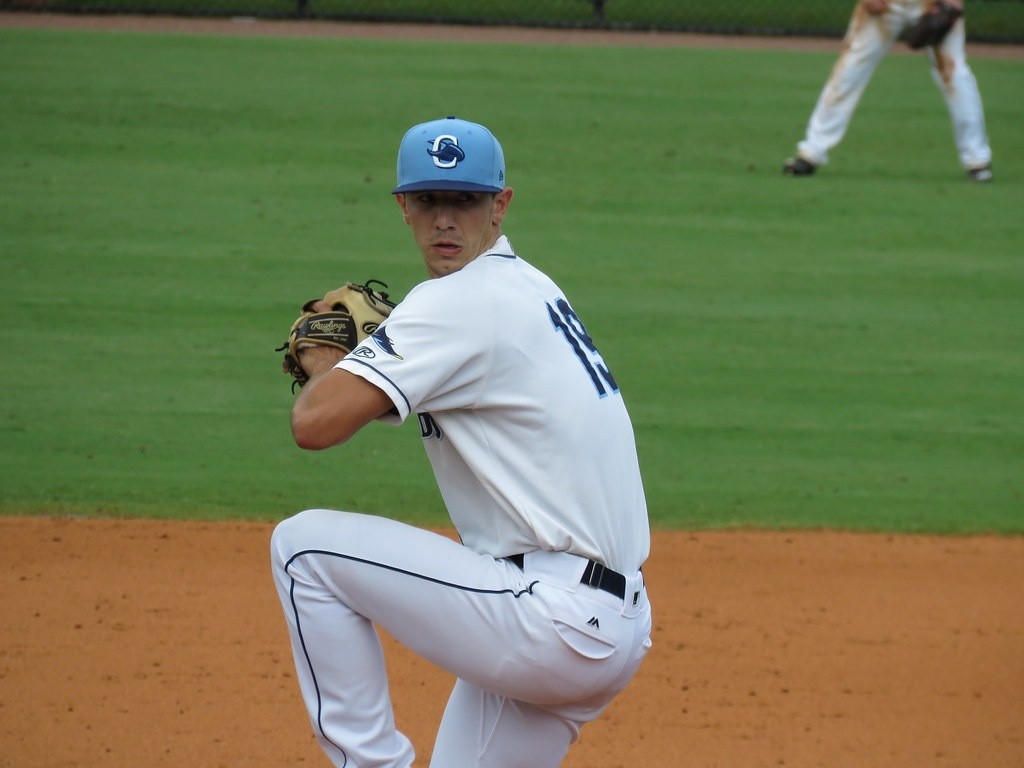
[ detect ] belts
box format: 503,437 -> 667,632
504,553 -> 645,603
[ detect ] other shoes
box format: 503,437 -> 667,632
969,164 -> 994,182
783,158 -> 815,177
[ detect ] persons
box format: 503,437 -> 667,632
270,116 -> 651,768
783,0 -> 993,181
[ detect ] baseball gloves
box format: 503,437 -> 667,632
899,4 -> 960,49
278,278 -> 399,390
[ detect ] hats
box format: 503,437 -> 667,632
392,115 -> 505,194
